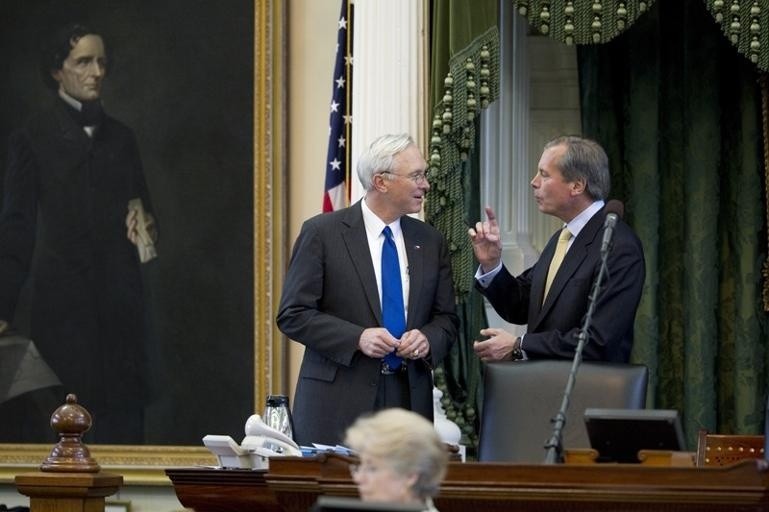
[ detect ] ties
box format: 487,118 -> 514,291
540,226 -> 574,311
379,226 -> 409,370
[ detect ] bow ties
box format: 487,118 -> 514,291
69,99 -> 108,127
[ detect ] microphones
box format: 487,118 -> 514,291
600,199 -> 625,255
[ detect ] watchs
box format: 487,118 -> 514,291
513,336 -> 523,362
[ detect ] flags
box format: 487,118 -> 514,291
322,2 -> 354,212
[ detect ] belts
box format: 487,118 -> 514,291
380,361 -> 409,375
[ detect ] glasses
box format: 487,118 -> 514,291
379,165 -> 432,187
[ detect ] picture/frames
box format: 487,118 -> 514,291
0,0 -> 292,490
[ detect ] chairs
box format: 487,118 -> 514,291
478,357 -> 650,462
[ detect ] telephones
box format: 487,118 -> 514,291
202,414 -> 303,470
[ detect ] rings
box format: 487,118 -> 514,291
413,350 -> 418,355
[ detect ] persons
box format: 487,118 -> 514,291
468,134 -> 645,366
343,407 -> 450,511
1,27 -> 158,444
276,131 -> 460,446
